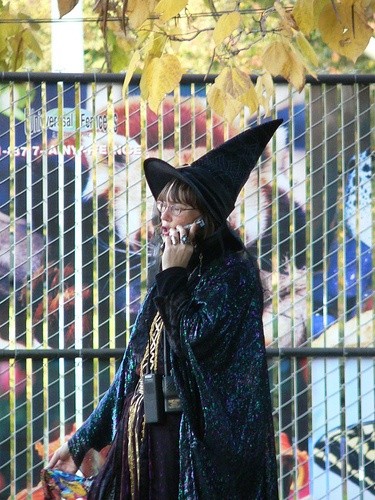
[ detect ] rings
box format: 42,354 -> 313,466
182,235 -> 188,243
171,235 -> 178,245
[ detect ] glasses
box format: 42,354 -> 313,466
155,200 -> 202,217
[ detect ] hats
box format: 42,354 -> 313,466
144,118 -> 284,252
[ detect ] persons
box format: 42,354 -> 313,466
44,118 -> 284,500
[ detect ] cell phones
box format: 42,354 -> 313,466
185,215 -> 207,237
